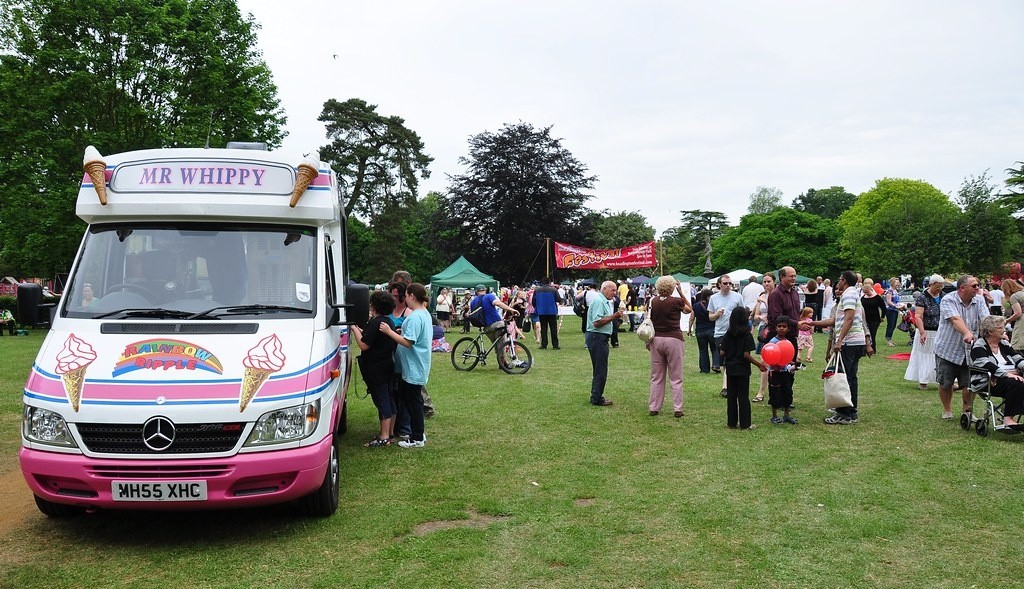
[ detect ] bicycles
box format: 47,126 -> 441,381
451,315 -> 532,375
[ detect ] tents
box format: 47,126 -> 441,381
672,273 -> 691,283
630,276 -> 649,285
689,276 -> 710,285
430,255 -> 595,316
643,275 -> 659,286
708,267 -> 762,291
741,270 -> 812,286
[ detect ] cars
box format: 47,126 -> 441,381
898,290 -> 914,301
925,282 -> 956,292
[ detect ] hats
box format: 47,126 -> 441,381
464,290 -> 470,294
476,284 -> 487,291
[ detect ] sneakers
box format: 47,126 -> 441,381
404,433 -> 426,441
824,414 -> 852,424
398,439 -> 424,448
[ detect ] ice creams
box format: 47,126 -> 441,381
83,146 -> 109,206
619,299 -> 626,313
290,151 -> 320,207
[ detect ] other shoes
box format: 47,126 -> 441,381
701,357 -> 812,429
941,411 -> 982,424
446,330 -> 450,333
553,346 -> 560,349
592,400 -> 612,405
499,363 -> 512,369
675,411 -> 684,417
539,346 -> 547,349
650,411 -> 658,415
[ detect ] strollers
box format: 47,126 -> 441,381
900,308 -> 918,347
572,301 -> 585,317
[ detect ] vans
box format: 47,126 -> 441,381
17,141 -> 370,518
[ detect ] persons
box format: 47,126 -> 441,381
80,283 -> 99,309
0,306 -> 16,336
350,266 -> 1024,449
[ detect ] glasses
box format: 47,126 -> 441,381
964,283 -> 979,288
721,282 -> 732,285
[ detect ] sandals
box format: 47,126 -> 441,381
364,437 -> 390,447
373,435 -> 395,444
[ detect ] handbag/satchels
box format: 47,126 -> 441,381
756,325 -> 769,344
824,350 -> 854,409
897,322 -> 909,331
469,295 -> 485,327
523,318 -> 531,332
637,296 -> 658,343
573,292 -> 586,318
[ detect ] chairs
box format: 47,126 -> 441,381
120,248 -> 177,310
243,230 -> 308,309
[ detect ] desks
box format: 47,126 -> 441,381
431,313 -> 461,328
557,306 -> 579,332
620,310 -> 645,325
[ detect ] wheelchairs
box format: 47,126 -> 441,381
960,339 -> 1024,437
